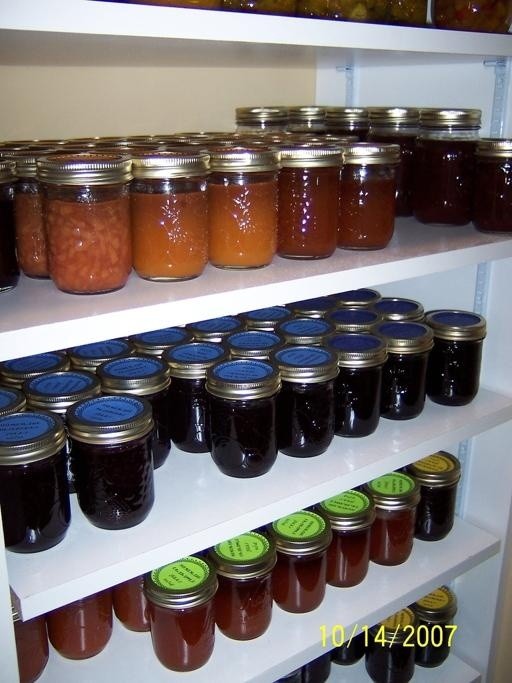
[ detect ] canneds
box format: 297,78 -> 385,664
138,0 -> 512,34
0,105 -> 512,295
0,290 -> 484,555
12,450 -> 461,683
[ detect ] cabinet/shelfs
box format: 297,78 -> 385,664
0,0 -> 511,682
